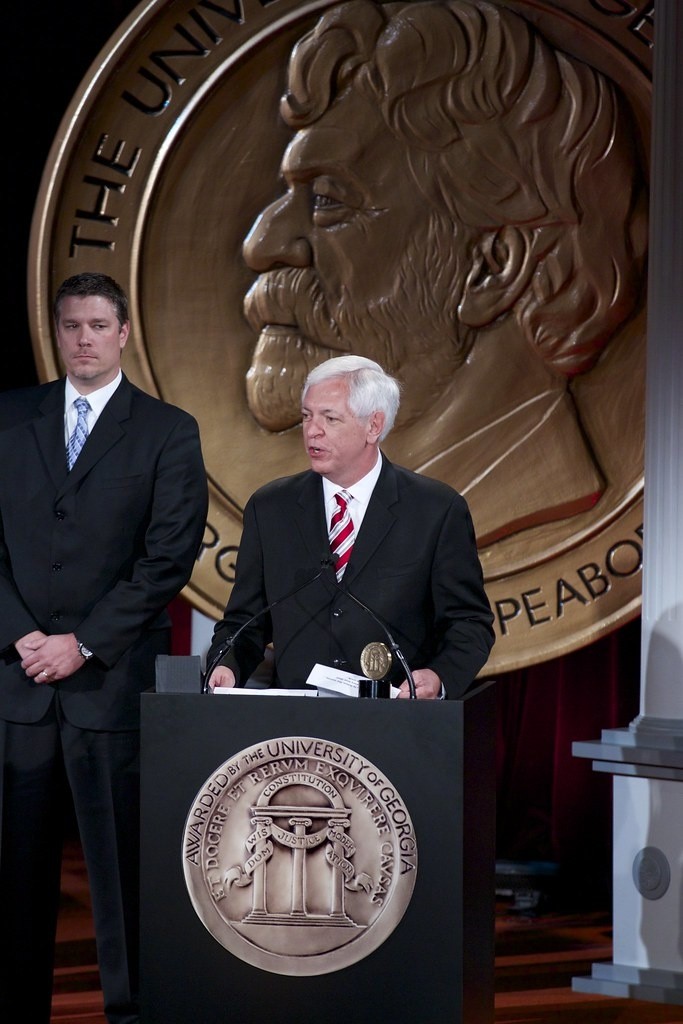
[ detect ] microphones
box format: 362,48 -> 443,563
203,557 -> 418,699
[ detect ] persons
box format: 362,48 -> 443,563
209,354 -> 496,698
2,274 -> 210,1023
240,0 -> 650,550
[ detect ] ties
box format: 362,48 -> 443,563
328,490 -> 355,583
66,398 -> 93,472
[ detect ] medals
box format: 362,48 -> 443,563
359,641 -> 392,680
